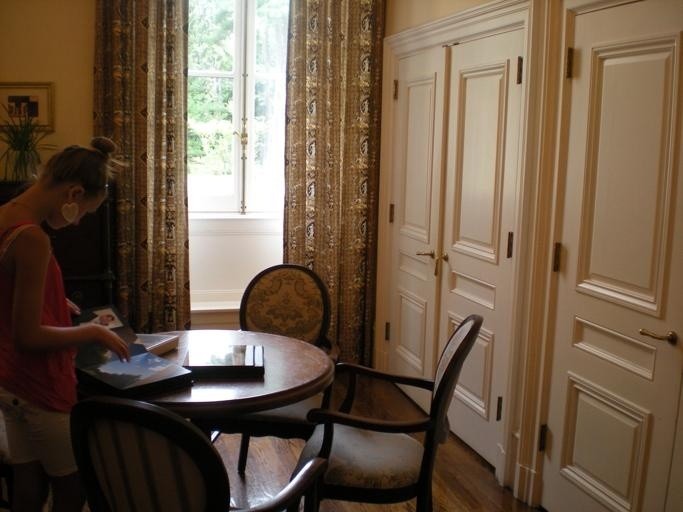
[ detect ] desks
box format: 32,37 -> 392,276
74,330 -> 335,448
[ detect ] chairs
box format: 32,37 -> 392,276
291,314 -> 484,512
69,394 -> 329,511
235,263 -> 344,475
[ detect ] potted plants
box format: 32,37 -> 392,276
0,105 -> 58,184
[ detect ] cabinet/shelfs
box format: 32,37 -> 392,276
0,180 -> 116,314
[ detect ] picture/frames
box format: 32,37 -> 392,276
0,82 -> 56,133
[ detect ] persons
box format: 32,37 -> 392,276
0,136 -> 130,512
